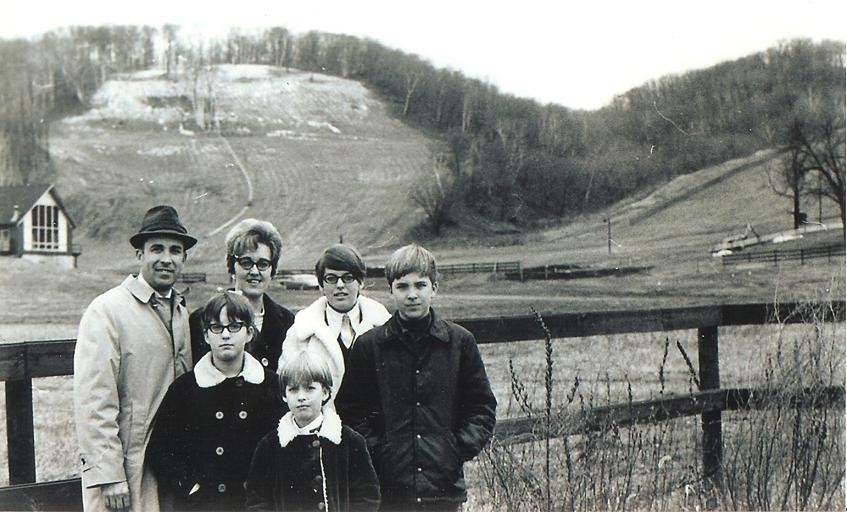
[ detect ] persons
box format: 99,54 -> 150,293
240,352 -> 381,511
74,205 -> 199,512
334,243 -> 498,511
276,243 -> 392,401
142,291 -> 289,511
190,217 -> 293,372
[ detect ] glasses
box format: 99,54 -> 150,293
323,273 -> 362,284
206,320 -> 249,333
234,253 -> 275,270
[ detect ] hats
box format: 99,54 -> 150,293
128,205 -> 198,251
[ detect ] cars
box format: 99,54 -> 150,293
280,273 -> 319,291
